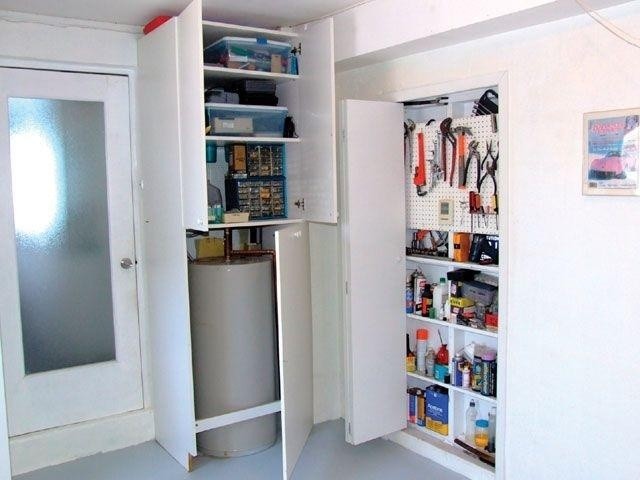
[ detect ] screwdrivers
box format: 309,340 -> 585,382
469,192 -> 499,233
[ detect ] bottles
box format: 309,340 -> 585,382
411,269 -> 470,390
465,399 -> 496,452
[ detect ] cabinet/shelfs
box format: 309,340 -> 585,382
339,86 -> 505,476
136,4 -> 342,476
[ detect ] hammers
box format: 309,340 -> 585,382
450,126 -> 473,189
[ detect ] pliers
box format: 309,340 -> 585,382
463,139 -> 499,192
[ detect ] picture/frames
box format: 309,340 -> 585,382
582,107 -> 640,196
